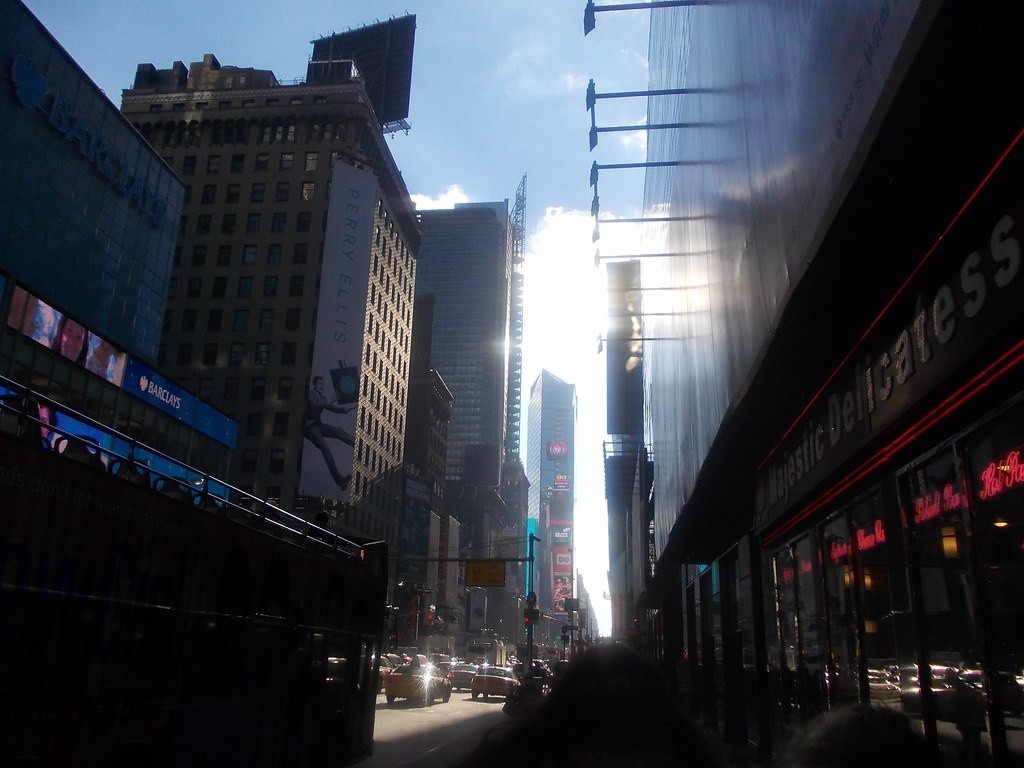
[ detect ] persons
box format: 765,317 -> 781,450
460,645 -> 724,768
48,316 -> 89,369
955,660 -> 988,768
305,512 -> 328,551
514,672 -> 544,711
250,500 -> 282,537
6,369 -> 55,446
779,703 -> 942,768
301,375 -> 355,491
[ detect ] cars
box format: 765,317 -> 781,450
362,651 -> 551,705
864,653 -> 1024,721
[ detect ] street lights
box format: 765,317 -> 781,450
526,534 -> 541,673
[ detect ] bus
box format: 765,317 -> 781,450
0,374 -> 394,768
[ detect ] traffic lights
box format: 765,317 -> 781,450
524,609 -> 531,627
427,610 -> 435,622
